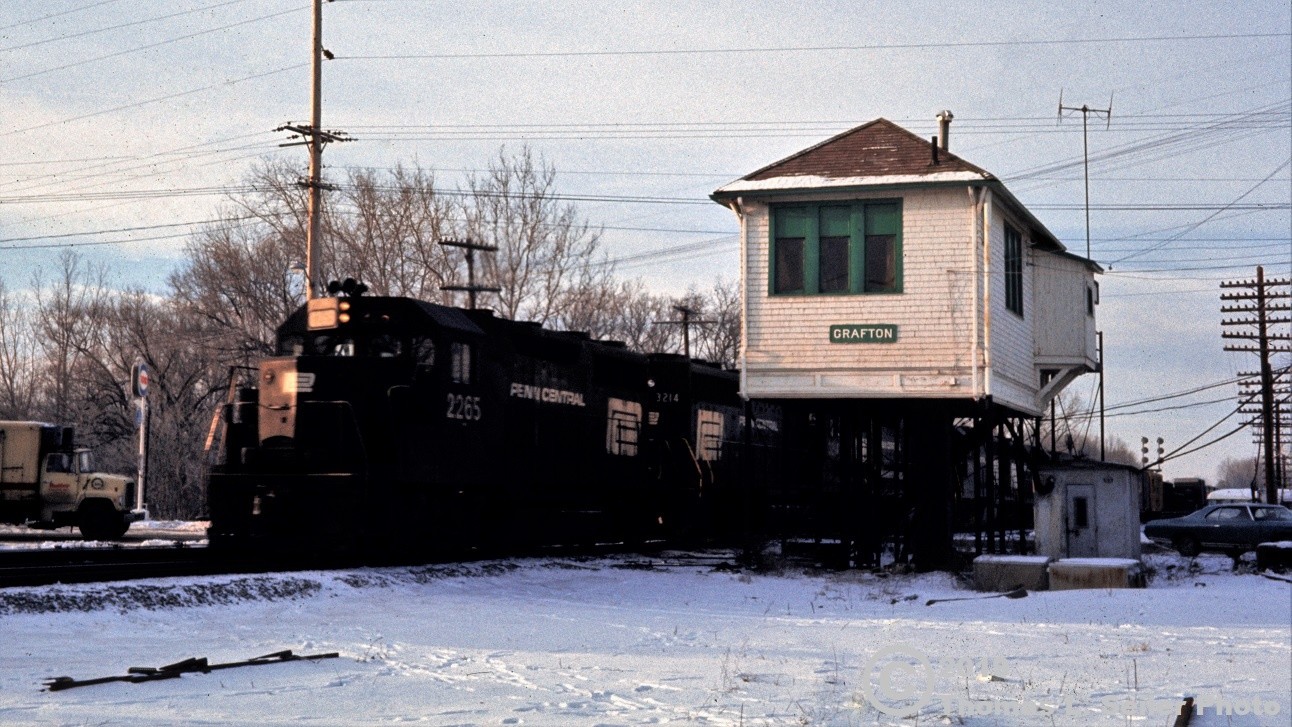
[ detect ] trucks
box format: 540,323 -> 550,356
0,420 -> 146,540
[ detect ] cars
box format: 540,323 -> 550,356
1143,501 -> 1292,559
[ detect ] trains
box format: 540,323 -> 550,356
201,280 -> 1164,562
